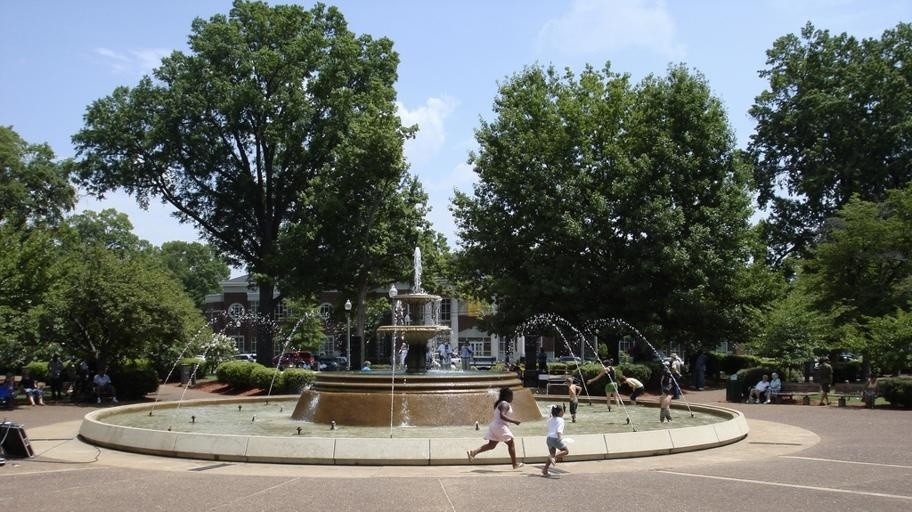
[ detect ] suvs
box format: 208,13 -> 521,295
272,349 -> 316,369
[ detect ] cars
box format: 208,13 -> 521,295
234,353 -> 256,361
839,354 -> 858,361
558,356 -> 583,361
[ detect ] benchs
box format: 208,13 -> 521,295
536,372 -> 572,392
776,380 -> 822,405
65,382 -> 117,404
469,355 -> 497,368
1,375 -> 48,405
826,383 -> 882,406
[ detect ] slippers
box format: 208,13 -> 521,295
467,451 -> 474,463
513,463 -> 524,470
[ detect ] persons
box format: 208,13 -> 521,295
619,374 -> 645,404
671,353 -> 682,399
817,355 -> 833,405
438,335 -> 454,371
764,371 -> 781,404
861,371 -> 881,408
658,388 -> 673,421
466,386 -> 524,470
537,345 -> 549,373
47,353 -> 64,400
565,377 -> 583,422
92,368 -> 120,404
360,360 -> 372,371
19,371 -> 46,406
746,374 -> 769,403
587,360 -> 619,407
541,405 -> 569,477
695,348 -> 708,390
460,336 -> 474,370
661,361 -> 673,397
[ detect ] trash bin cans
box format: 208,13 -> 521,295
726,374 -> 742,402
179,363 -> 191,384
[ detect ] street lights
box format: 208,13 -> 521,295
388,285 -> 398,368
344,296 -> 353,370
